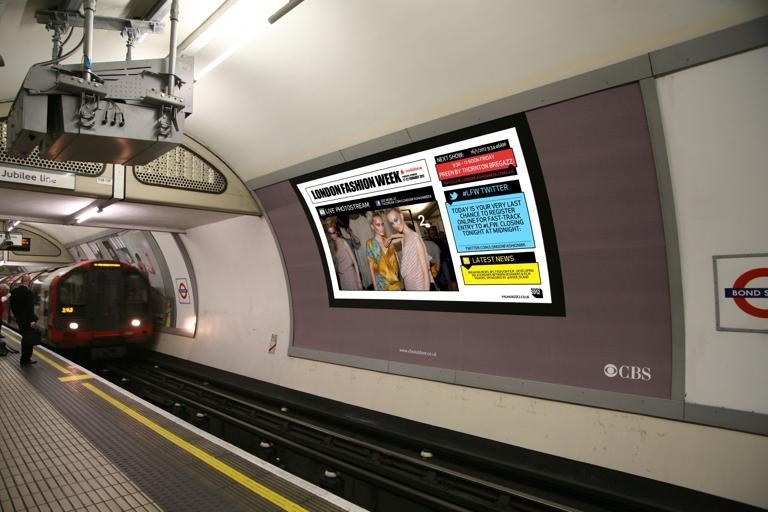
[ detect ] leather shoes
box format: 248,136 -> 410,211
23,358 -> 37,366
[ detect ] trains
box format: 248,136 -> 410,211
0,258 -> 159,368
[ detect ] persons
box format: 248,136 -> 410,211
365,213 -> 403,290
388,207 -> 431,290
11,274 -> 39,366
323,217 -> 363,290
336,221 -> 360,250
430,229 -> 451,290
0,284 -> 10,338
439,231 -> 455,277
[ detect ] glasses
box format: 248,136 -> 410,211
328,231 -> 336,235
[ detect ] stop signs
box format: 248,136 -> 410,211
178,283 -> 188,299
724,265 -> 768,320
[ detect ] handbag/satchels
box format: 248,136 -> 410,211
22,327 -> 41,346
